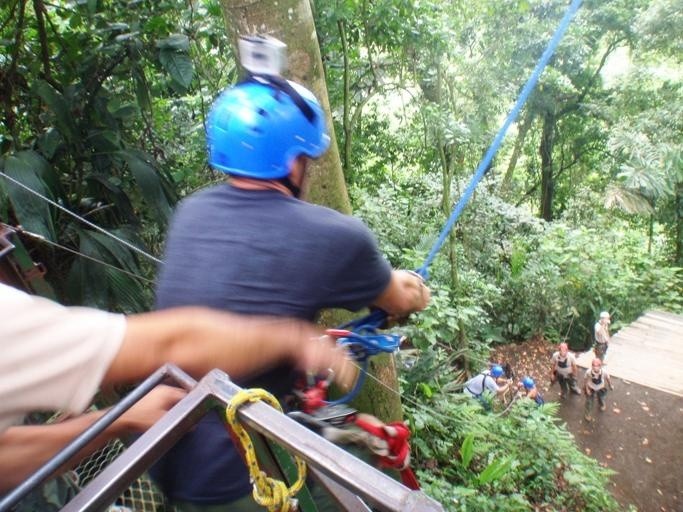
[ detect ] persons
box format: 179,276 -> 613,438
462,364 -> 512,409
547,341 -> 580,399
581,357 -> 607,407
518,376 -> 537,405
144,68 -> 429,511
1,277 -> 359,491
593,311 -> 611,366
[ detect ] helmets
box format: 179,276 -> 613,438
492,367 -> 503,376
523,377 -> 533,387
600,312 -> 608,317
206,78 -> 330,178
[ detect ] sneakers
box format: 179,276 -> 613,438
560,392 -> 566,399
600,403 -> 605,411
571,386 -> 582,394
585,415 -> 591,422
